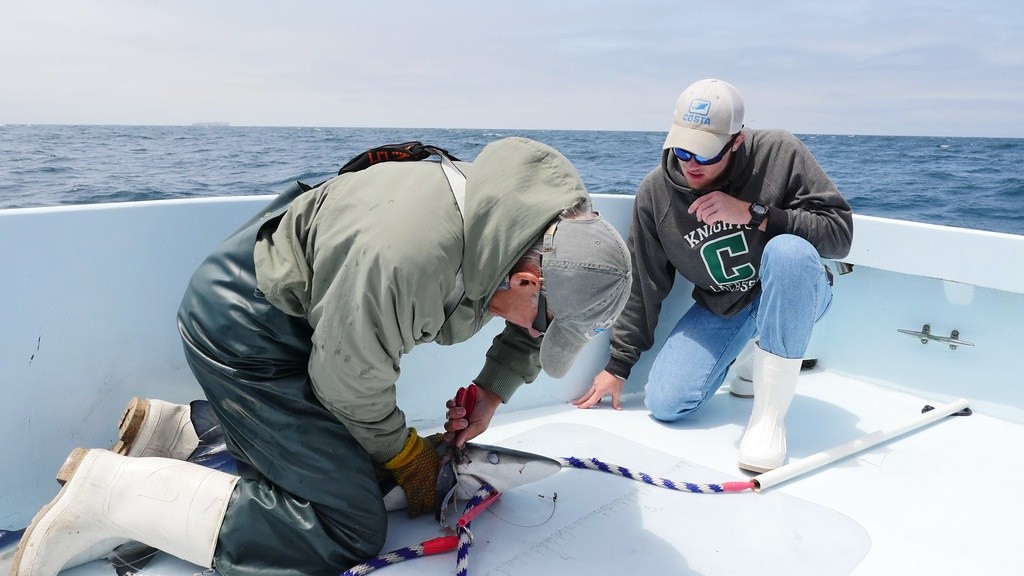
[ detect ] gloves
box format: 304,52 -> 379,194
381,426 -> 445,518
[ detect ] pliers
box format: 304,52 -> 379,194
434,383 -> 477,520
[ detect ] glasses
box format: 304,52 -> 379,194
532,294 -> 547,334
671,125 -> 743,166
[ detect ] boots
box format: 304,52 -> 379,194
730,336 -> 762,398
10,446 -> 240,576
113,398 -> 199,461
737,340 -> 803,473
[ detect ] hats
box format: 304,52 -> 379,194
663,79 -> 745,160
540,210 -> 633,379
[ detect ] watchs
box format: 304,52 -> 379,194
745,200 -> 768,230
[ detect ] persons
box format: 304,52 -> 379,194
9,133 -> 634,576
572,78 -> 854,476
